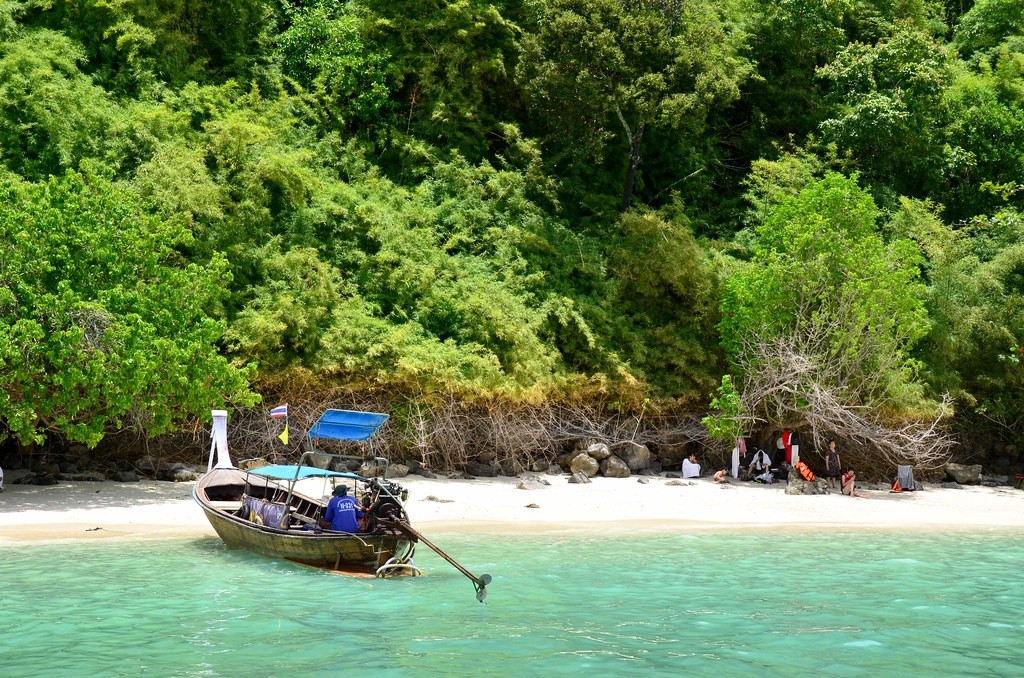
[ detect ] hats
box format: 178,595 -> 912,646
332,485 -> 348,497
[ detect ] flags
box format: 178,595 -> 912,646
269,402 -> 287,419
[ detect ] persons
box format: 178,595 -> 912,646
825,441 -> 841,489
712,467 -> 729,484
839,466 -> 872,499
323,484 -> 365,535
681,453 -> 700,478
746,449 -> 772,479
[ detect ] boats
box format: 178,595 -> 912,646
192,408 -> 417,578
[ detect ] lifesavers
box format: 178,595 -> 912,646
241,505 -> 249,519
